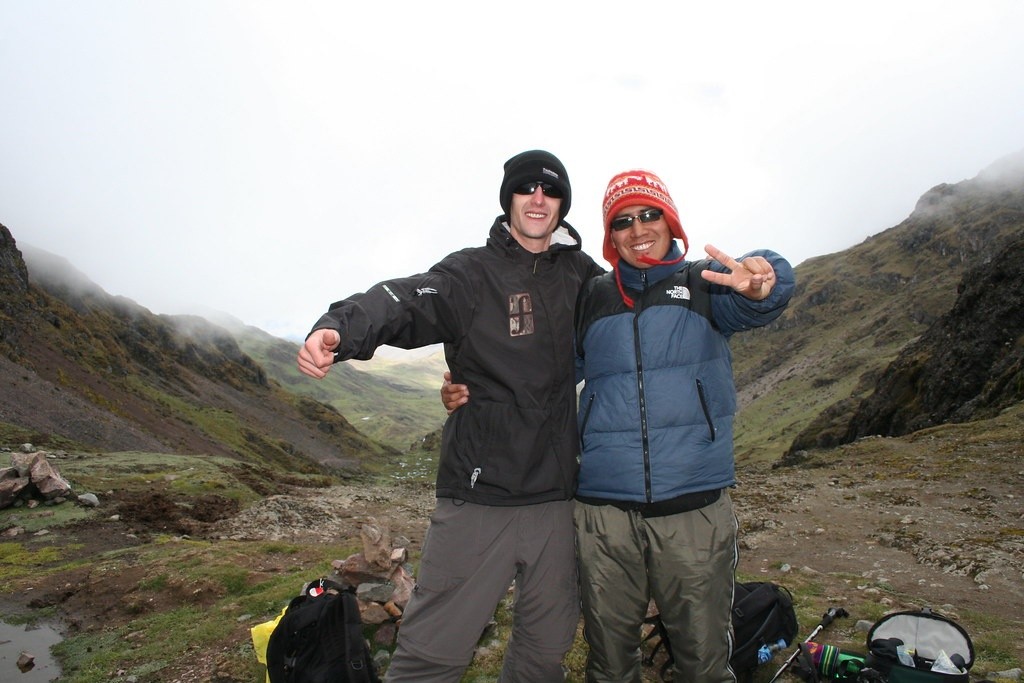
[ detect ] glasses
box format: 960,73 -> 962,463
610,209 -> 664,231
512,180 -> 562,198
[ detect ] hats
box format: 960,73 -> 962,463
499,150 -> 572,218
602,170 -> 689,307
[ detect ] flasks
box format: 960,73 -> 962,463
806,642 -> 865,676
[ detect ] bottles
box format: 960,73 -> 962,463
756,639 -> 788,665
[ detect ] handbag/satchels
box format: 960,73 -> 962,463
865,606 -> 975,683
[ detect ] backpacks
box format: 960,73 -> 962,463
267,578 -> 382,683
640,581 -> 798,678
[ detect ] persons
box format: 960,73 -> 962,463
296,149 -> 796,683
